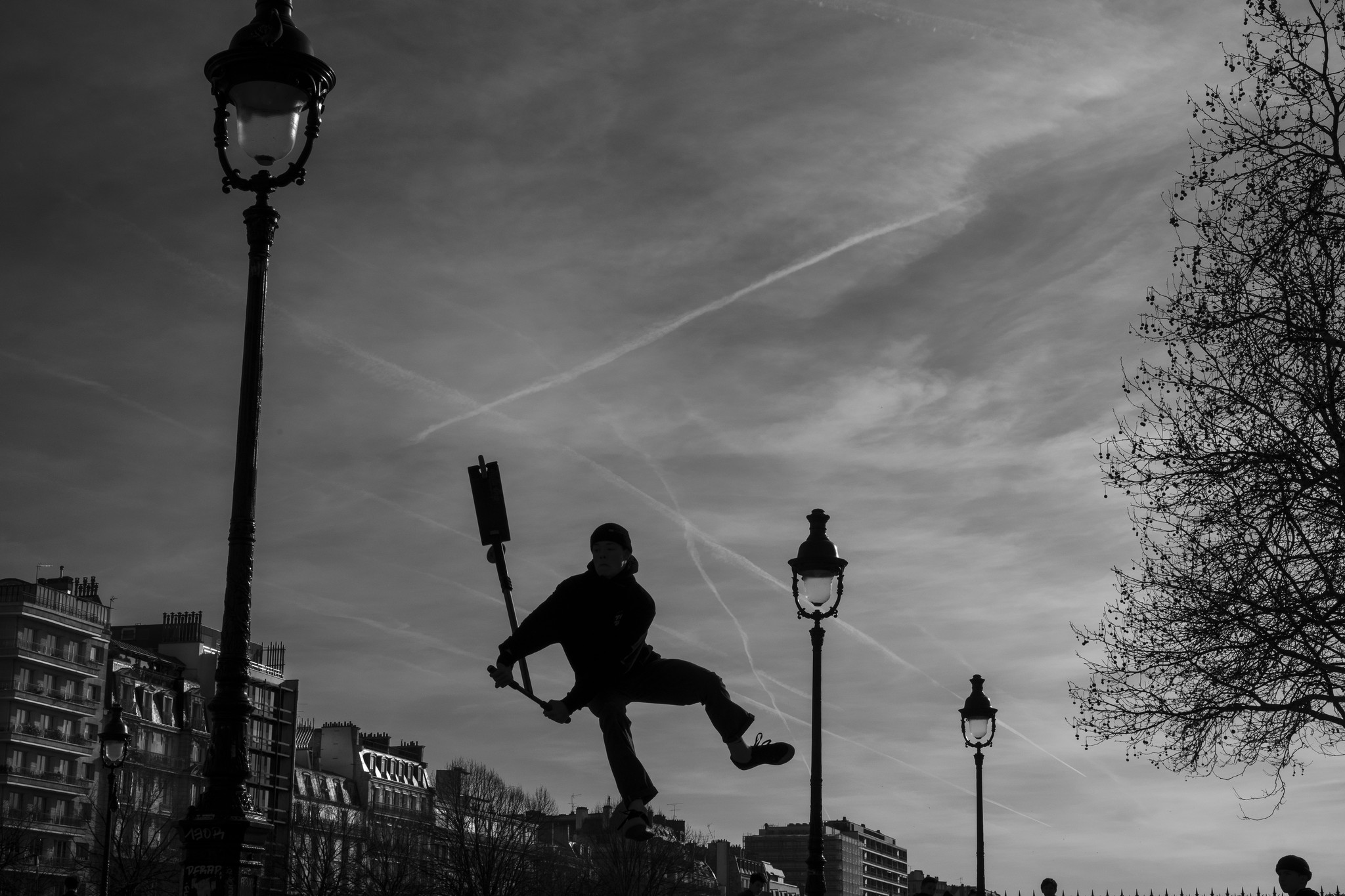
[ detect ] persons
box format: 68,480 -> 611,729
1040,877 -> 1057,896
493,521 -> 796,823
1276,855 -> 1321,896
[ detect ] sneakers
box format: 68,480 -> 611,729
618,807 -> 654,841
730,732 -> 795,770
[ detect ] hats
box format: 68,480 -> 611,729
590,522 -> 632,555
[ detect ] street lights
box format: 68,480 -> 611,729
957,674 -> 998,896
195,0 -> 337,896
96,703 -> 135,896
787,507 -> 849,896
